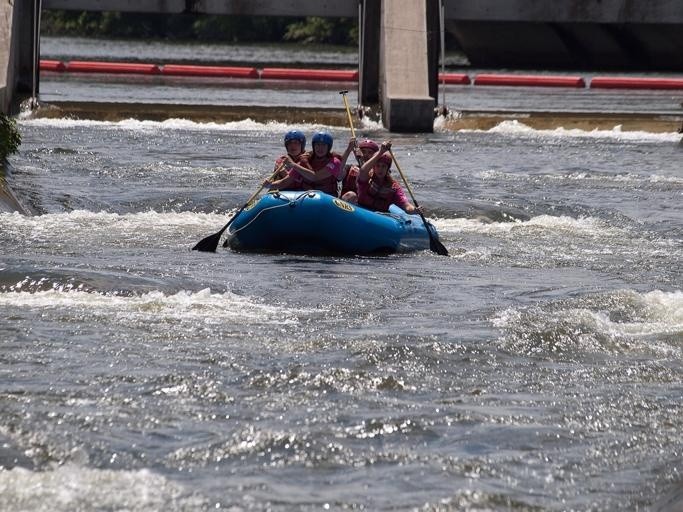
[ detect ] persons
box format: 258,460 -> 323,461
261,130 -> 424,214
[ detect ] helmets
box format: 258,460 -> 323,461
312,131 -> 333,146
284,129 -> 305,148
379,153 -> 392,166
358,140 -> 379,151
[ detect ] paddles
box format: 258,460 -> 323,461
192,157 -> 289,252
387,147 -> 450,257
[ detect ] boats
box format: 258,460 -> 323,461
228,189 -> 439,255
